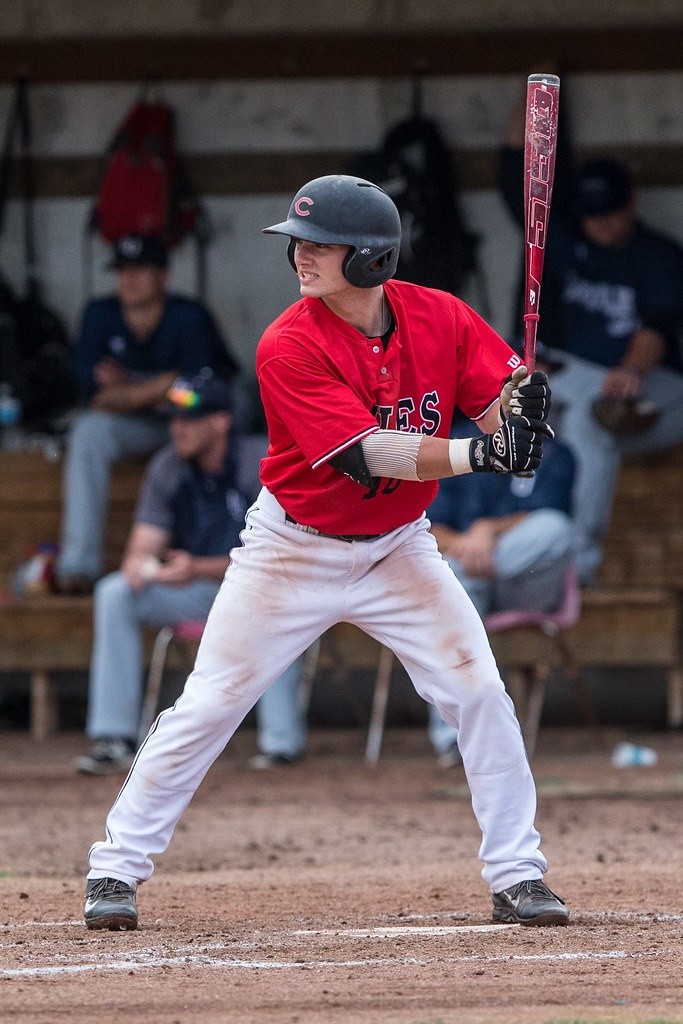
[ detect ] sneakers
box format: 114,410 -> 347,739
491,876 -> 567,928
85,875 -> 136,931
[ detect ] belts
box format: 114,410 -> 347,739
289,513 -> 394,543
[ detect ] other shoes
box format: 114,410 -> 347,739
253,748 -> 300,770
79,737 -> 134,773
607,398 -> 663,437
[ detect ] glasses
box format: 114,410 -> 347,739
162,387 -> 202,411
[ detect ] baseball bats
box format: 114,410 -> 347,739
512,70 -> 564,478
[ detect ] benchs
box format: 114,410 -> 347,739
0,443 -> 682,762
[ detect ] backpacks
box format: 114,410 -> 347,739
373,115 -> 468,294
88,71 -> 200,249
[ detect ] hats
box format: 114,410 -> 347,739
567,158 -> 630,216
147,370 -> 234,420
107,237 -> 167,267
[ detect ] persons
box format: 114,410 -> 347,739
48,232 -> 245,594
74,373 -> 307,773
85,174 -> 569,933
533,156 -> 683,590
428,348 -> 576,768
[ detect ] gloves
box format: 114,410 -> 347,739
499,366 -> 551,423
470,420 -> 540,478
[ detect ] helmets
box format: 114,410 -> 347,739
259,171 -> 402,290
